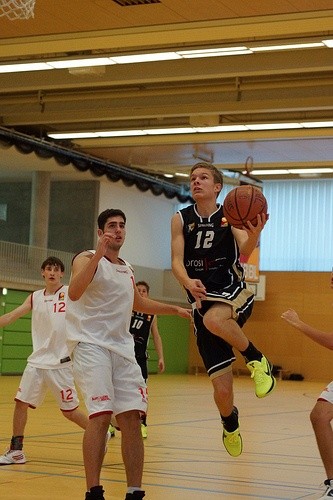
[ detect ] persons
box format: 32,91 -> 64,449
63,209 -> 194,500
280,267 -> 333,500
171,162 -> 277,457
0,255 -> 111,464
107,281 -> 165,438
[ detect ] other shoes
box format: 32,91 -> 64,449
125,491 -> 145,500
85,486 -> 105,500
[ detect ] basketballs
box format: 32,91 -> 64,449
223,185 -> 268,230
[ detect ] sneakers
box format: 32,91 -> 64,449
0,450 -> 26,464
220,406 -> 242,457
104,430 -> 111,455
140,415 -> 148,438
245,353 -> 276,398
107,422 -> 115,435
320,479 -> 333,500
